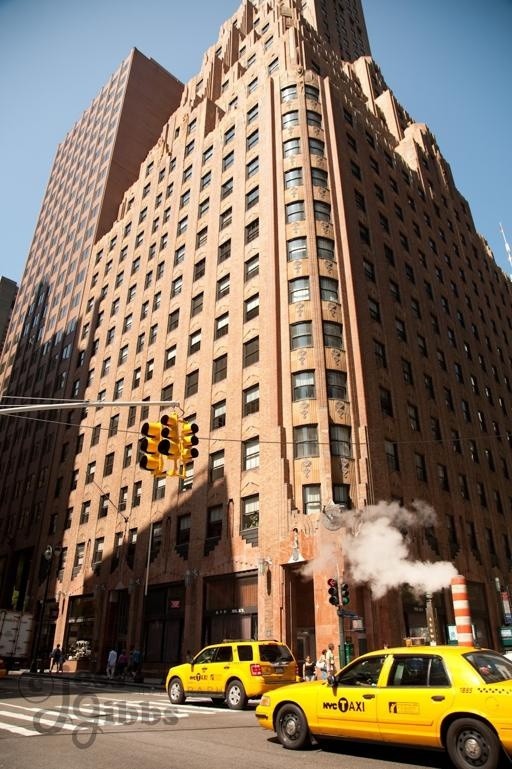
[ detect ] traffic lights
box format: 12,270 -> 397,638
327,578 -> 350,605
139,412 -> 199,480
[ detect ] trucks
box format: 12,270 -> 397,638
0,609 -> 36,669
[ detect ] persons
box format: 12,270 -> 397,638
287,528 -> 305,563
357,679 -> 377,687
383,641 -> 391,649
186,648 -> 194,663
303,655 -> 318,681
106,646 -> 144,683
319,643 -> 335,680
48,644 -> 66,674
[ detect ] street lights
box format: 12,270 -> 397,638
30,545 -> 54,674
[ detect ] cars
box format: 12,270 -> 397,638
165,640 -> 511,769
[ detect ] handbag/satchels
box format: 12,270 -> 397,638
316,660 -> 325,669
